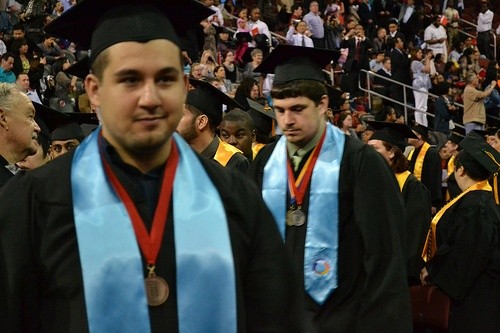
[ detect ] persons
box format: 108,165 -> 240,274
0,0 -> 285,333
243,43 -> 411,333
0,0 -> 500,284
419,135 -> 500,333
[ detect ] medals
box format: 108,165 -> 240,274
284,205 -> 296,227
141,264 -> 169,306
292,204 -> 305,226
